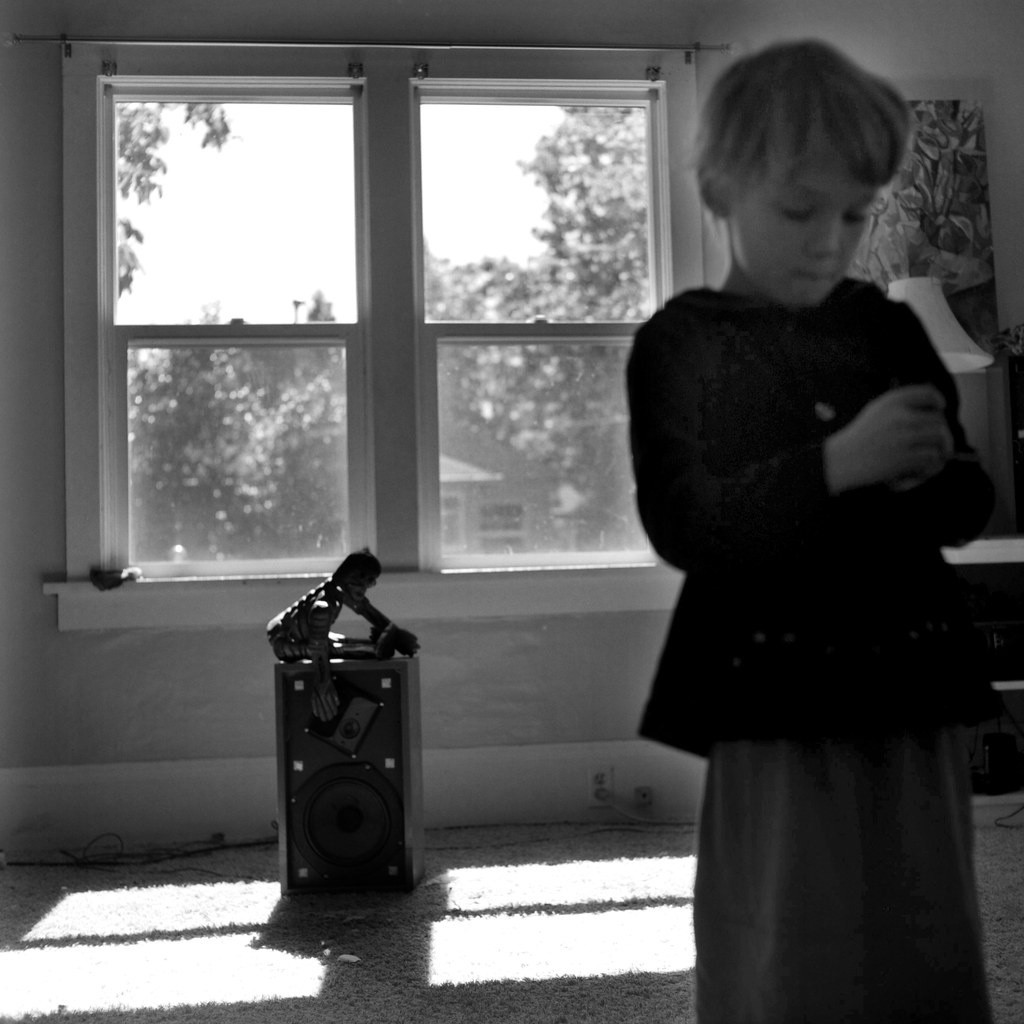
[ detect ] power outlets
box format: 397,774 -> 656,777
588,765 -> 613,807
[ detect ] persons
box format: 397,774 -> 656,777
624,37 -> 999,1021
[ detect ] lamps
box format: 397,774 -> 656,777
888,276 -> 993,373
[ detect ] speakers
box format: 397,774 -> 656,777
272,655 -> 424,897
